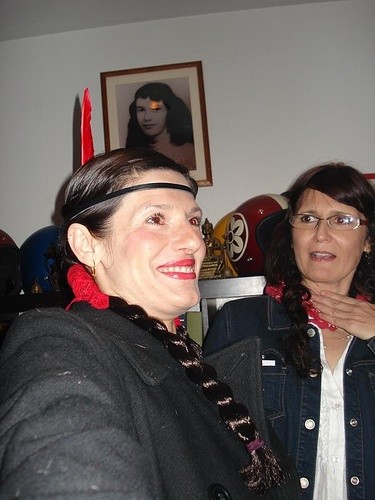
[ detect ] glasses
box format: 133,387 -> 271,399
288,211 -> 369,230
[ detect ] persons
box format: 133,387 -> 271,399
200,162 -> 375,500
1,148 -> 292,500
125,82 -> 197,170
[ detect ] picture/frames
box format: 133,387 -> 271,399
99,60 -> 215,188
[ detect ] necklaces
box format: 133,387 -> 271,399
324,334 -> 352,349
